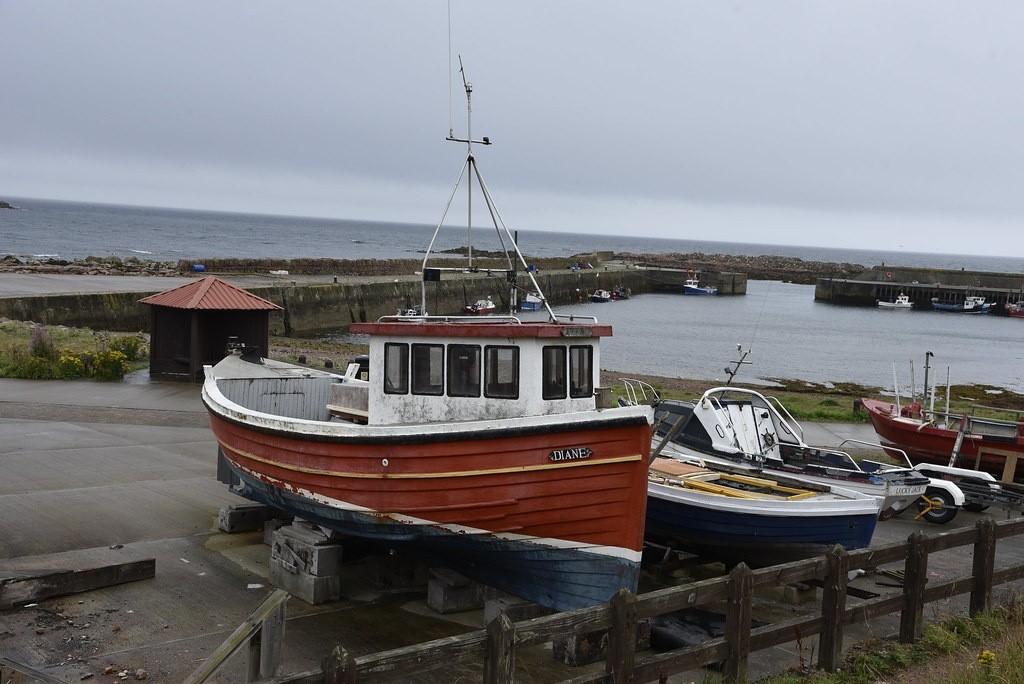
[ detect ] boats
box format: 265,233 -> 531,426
1005,282 -> 1024,318
588,289 -> 611,302
683,272 -> 718,294
931,292 -> 997,314
609,283 -> 632,299
644,426 -> 886,566
198,48 -> 661,623
518,289 -> 544,311
392,306 -> 429,323
860,347 -> 1024,487
876,290 -> 916,309
612,340 -> 931,523
466,295 -> 496,316
